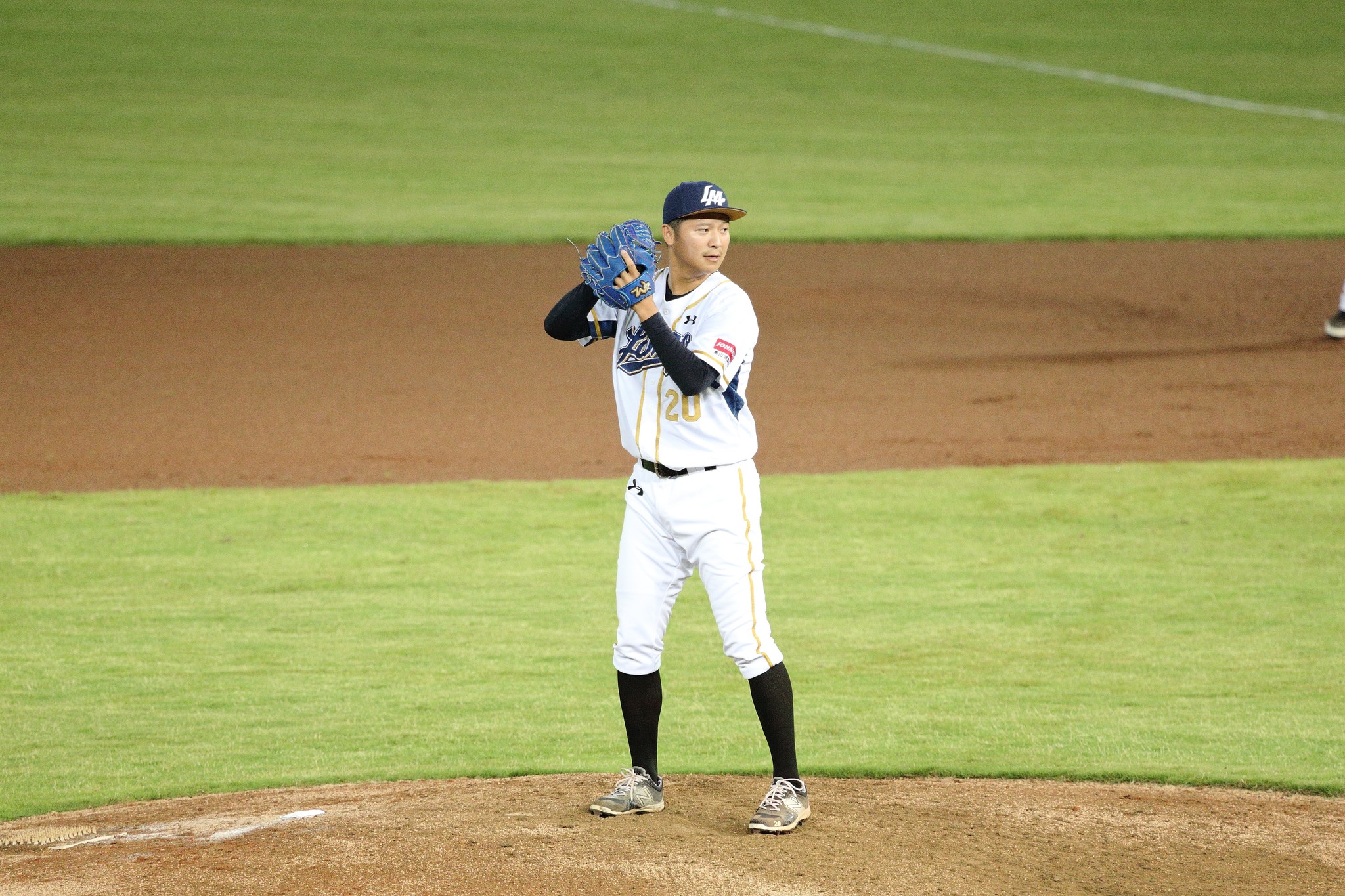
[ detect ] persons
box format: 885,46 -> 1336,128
543,180 -> 812,832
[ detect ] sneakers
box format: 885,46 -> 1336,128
590,766 -> 665,816
748,776 -> 811,836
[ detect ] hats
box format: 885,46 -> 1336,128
662,179 -> 747,226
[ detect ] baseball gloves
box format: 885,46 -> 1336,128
579,219 -> 656,311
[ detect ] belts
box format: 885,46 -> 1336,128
641,459 -> 717,480
